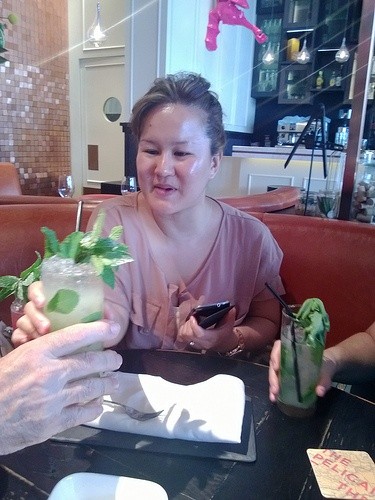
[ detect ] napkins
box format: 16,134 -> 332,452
80,371 -> 246,444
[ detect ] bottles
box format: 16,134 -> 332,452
330,64 -> 344,88
316,71 -> 324,89
332,107 -> 352,150
257,18 -> 282,92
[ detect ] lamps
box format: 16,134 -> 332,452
262,0 -> 276,65
335,0 -> 350,63
88,0 -> 110,49
298,0 -> 311,65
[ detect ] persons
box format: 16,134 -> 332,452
268,321 -> 375,403
0,317 -> 123,456
10,70 -> 286,360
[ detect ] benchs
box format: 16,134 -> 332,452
0,162 -> 375,405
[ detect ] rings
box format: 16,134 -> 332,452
3,325 -> 13,337
189,341 -> 195,346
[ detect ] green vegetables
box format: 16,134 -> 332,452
297,297 -> 330,366
0,208 -> 135,322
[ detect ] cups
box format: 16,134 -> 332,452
58,175 -> 75,198
278,305 -> 325,418
40,257 -> 105,381
121,175 -> 137,196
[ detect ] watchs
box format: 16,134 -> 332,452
217,326 -> 247,360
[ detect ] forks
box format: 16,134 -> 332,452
104,400 -> 164,421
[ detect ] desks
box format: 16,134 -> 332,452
232,145 -> 375,196
0,350 -> 375,500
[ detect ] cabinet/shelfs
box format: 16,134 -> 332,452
250,0 -> 375,107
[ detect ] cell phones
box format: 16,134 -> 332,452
185,300 -> 231,323
